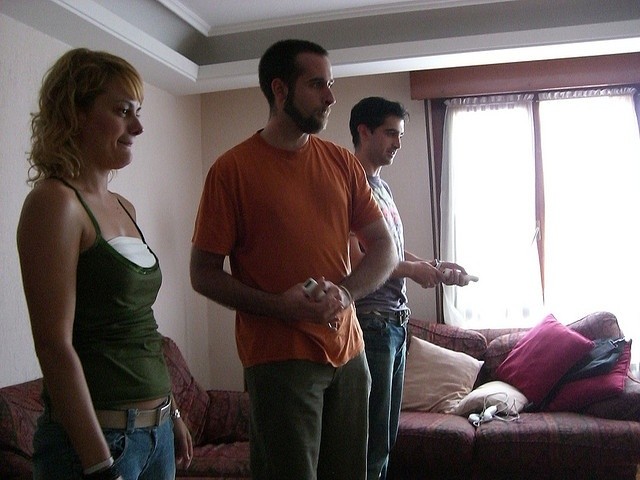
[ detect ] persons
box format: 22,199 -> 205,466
17,48 -> 194,479
348,96 -> 468,480
189,38 -> 399,480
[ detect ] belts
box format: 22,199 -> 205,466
380,310 -> 410,326
97,398 -> 172,432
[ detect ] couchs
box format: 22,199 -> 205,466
0,377 -> 249,480
396,319 -> 639,480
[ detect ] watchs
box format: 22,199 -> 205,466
170,410 -> 182,421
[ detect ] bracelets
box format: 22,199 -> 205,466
82,457 -> 116,477
339,285 -> 354,304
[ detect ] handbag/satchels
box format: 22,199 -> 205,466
561,336 -> 626,382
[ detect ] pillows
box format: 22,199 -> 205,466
493,314 -> 596,404
453,381 -> 527,415
157,335 -> 211,449
405,317 -> 487,384
549,340 -> 632,413
398,336 -> 485,413
488,311 -> 624,380
